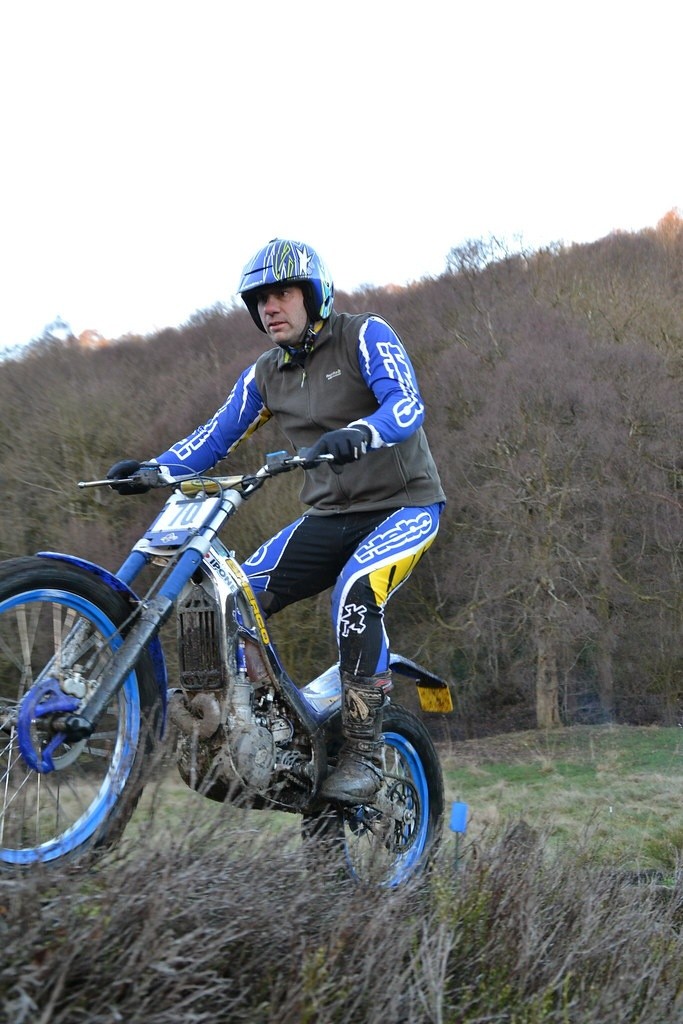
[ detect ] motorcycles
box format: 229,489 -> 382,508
0,451 -> 460,912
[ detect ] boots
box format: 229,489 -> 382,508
321,667 -> 394,804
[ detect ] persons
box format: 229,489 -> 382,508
106,236 -> 448,804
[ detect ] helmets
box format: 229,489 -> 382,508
235,238 -> 334,333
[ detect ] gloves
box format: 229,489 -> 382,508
106,460 -> 161,495
306,424 -> 373,466
